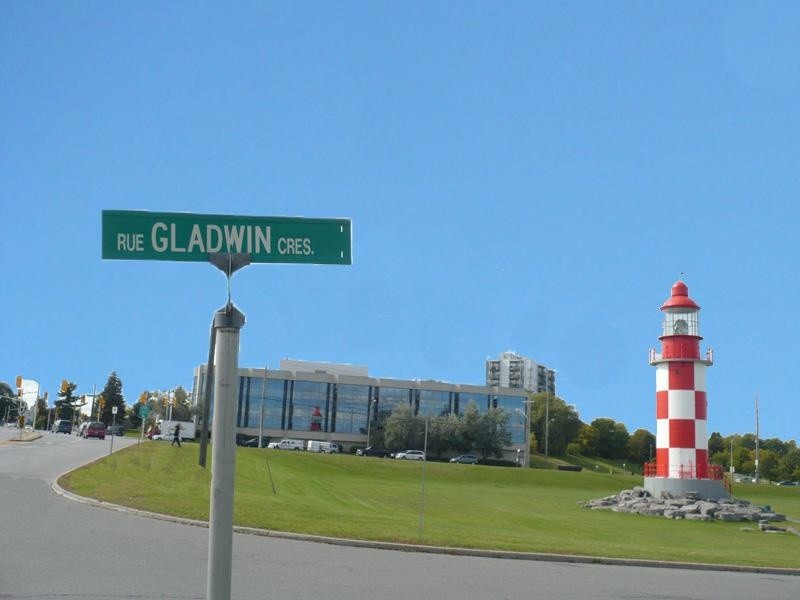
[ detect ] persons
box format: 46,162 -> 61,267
170,423 -> 182,447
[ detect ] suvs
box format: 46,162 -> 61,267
268,439 -> 304,452
396,450 -> 425,462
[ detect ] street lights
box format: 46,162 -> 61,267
515,408 -> 527,468
544,418 -> 555,470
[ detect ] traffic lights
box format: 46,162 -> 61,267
162,396 -> 167,408
173,397 -> 176,408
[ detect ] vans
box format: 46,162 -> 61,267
105,426 -> 125,435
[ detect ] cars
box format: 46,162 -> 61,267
356,446 -> 385,458
51,419 -> 72,434
235,434 -> 250,446
152,432 -> 183,442
735,476 -> 752,484
83,421 -> 106,441
449,455 -> 481,465
778,480 -> 796,486
76,422 -> 88,438
242,437 -> 269,449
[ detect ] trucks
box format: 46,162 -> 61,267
146,419 -> 196,441
307,440 -> 340,455
